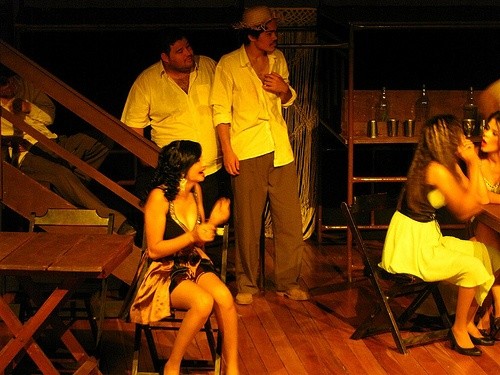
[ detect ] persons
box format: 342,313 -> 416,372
128,140 -> 240,375
121,35 -> 233,225
212,7 -> 308,304
0,67 -> 137,234
473,110 -> 500,345
378,112 -> 494,355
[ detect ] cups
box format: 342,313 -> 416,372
368,120 -> 377,138
404,119 -> 415,137
463,119 -> 475,137
387,118 -> 398,137
475,120 -> 485,137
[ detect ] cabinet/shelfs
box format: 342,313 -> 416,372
340,89 -> 481,229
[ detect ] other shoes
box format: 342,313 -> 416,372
117,223 -> 136,236
236,291 -> 253,305
468,331 -> 495,346
276,288 -> 310,301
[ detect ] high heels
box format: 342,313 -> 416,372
448,325 -> 482,355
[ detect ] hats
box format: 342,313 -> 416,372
232,5 -> 283,30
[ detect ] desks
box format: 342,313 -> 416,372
0,232 -> 133,375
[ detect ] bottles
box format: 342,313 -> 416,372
414,85 -> 431,122
376,87 -> 389,122
463,86 -> 477,119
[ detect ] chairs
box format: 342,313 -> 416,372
14,207 -> 114,361
131,224 -> 229,375
340,201 -> 453,355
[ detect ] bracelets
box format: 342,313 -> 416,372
193,230 -> 204,244
